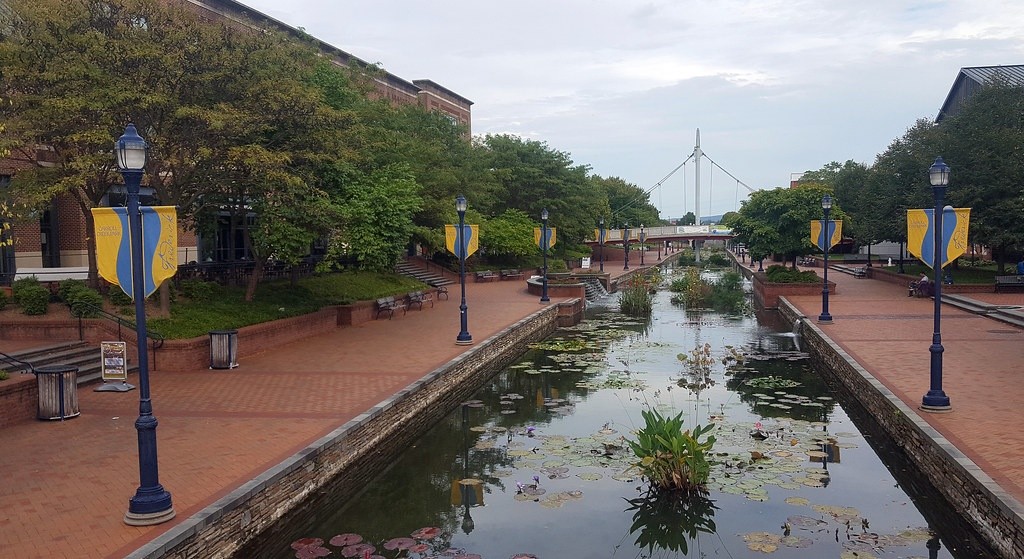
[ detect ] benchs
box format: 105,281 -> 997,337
853,266 -> 868,279
908,278 -> 932,298
375,296 -> 408,321
994,275 -> 1024,294
406,290 -> 435,311
500,269 -> 525,280
424,286 -> 450,301
799,257 -> 815,267
476,271 -> 499,282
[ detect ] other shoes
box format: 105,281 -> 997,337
907,287 -> 914,291
908,294 -> 913,297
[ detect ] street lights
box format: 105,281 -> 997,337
640,223 -> 645,265
112,122 -> 178,527
462,404 -> 475,536
624,219 -> 629,269
818,194 -> 833,320
454,190 -> 474,345
540,207 -> 549,301
922,155 -> 952,407
600,218 -> 605,273
819,410 -> 831,488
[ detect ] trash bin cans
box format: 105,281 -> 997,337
34,364 -> 81,421
567,257 -> 582,269
209,329 -> 239,369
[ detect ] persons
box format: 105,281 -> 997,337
907,272 -> 929,296
